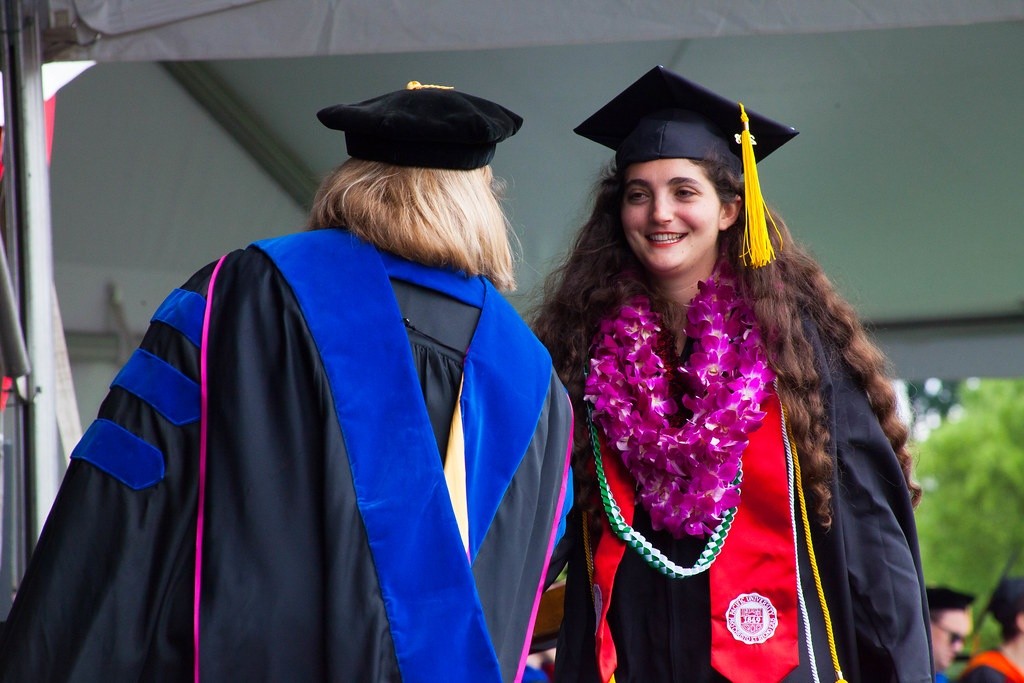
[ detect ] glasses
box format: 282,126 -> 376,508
935,624 -> 968,647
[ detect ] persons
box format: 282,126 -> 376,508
521,645 -> 556,683
526,66 -> 936,683
958,577 -> 1024,683
0,83 -> 576,683
926,583 -> 978,683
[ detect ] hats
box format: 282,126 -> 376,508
316,80 -> 524,171
573,65 -> 800,271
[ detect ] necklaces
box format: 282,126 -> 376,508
591,444 -> 742,577
582,275 -> 773,536
